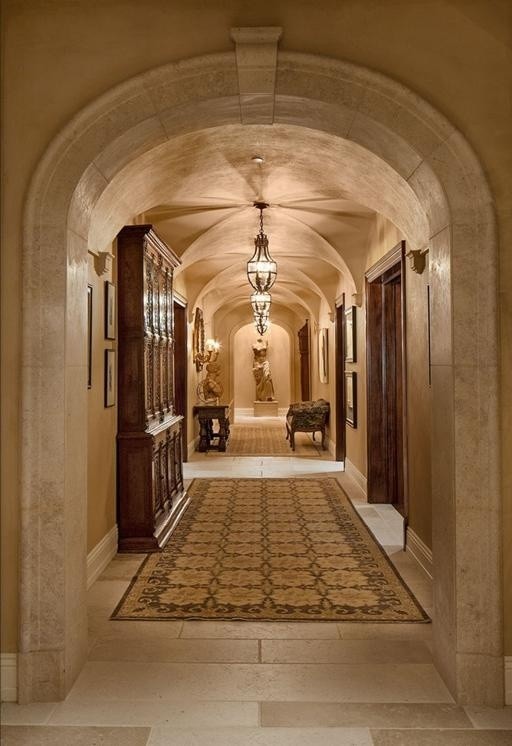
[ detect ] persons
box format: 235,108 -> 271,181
252,336 -> 277,402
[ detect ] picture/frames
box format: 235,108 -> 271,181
86,282 -> 94,390
104,279 -> 117,342
317,327 -> 330,385
103,348 -> 117,409
341,305 -> 360,364
342,370 -> 359,430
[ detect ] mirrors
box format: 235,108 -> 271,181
192,306 -> 208,373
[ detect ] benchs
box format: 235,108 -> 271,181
285,397 -> 330,452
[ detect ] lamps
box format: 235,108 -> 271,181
196,338 -> 220,363
244,200 -> 280,339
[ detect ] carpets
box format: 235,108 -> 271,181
205,418 -> 322,458
107,476 -> 433,625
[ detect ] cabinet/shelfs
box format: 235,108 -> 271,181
116,222 -> 193,554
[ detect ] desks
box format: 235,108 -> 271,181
192,399 -> 234,454
